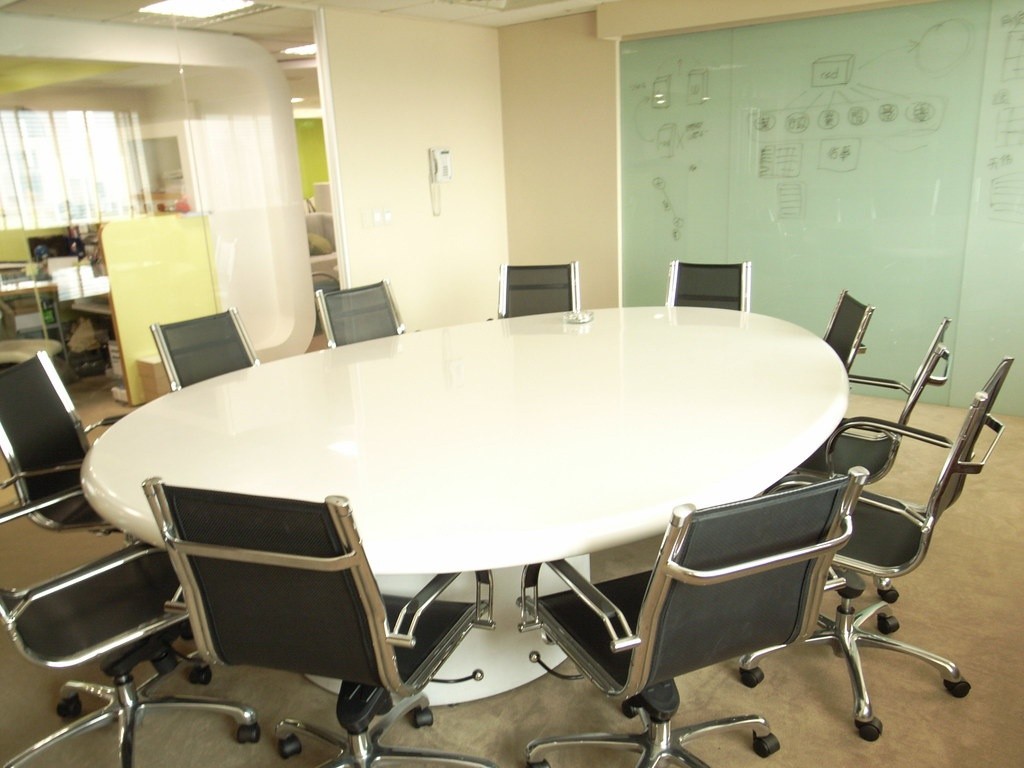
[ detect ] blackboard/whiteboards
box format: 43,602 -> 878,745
610,0 -> 1024,423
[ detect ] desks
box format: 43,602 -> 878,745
72,303 -> 110,315
81,307 -> 849,706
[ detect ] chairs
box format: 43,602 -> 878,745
0,340 -> 63,365
737,355 -> 1015,741
0,485 -> 261,768
315,279 -> 405,348
0,351 -> 142,547
149,307 -> 260,393
142,477 -> 503,768
517,465 -> 869,768
823,289 -> 876,374
498,261 -> 580,318
666,260 -> 751,312
789,318 -> 953,485
